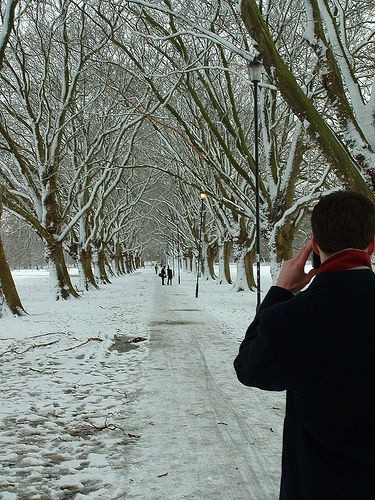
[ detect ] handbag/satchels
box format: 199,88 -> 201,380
159,273 -> 166,278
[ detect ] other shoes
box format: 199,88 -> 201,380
162,284 -> 165,285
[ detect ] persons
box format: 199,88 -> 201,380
233,189 -> 375,500
152,263 -> 173,285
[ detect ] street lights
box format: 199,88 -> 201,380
247,55 -> 263,315
196,192 -> 206,298
176,218 -> 181,284
